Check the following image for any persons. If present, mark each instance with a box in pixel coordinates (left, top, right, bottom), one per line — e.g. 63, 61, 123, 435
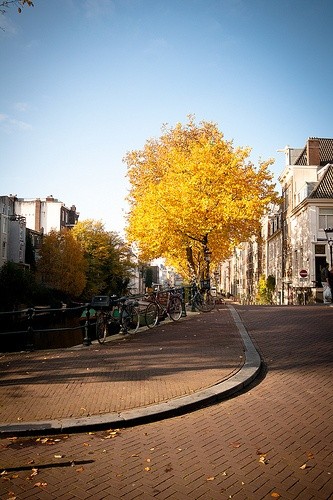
318, 263, 333, 304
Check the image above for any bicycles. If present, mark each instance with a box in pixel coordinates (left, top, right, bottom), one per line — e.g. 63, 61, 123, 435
144, 284, 183, 329
187, 282, 215, 312
91, 294, 141, 344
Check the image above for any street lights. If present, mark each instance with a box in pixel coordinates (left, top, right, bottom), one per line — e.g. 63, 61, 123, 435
323, 227, 333, 270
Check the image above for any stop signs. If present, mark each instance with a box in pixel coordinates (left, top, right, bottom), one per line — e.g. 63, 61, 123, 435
300, 270, 308, 277
